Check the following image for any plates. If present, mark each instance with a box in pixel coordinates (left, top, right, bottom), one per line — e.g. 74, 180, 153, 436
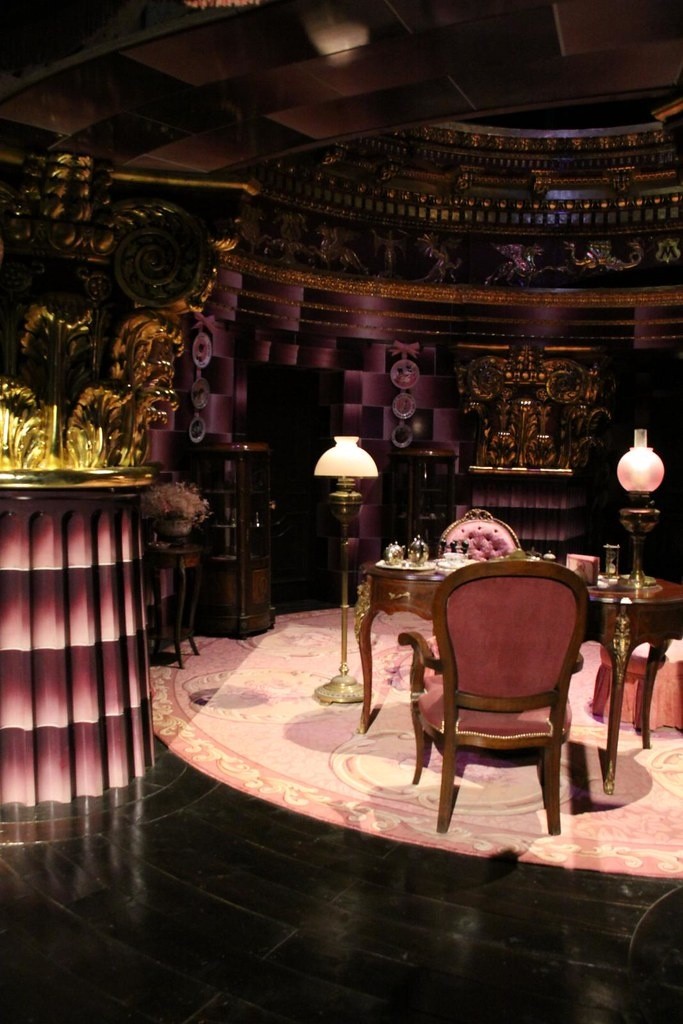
193, 333, 212, 368
391, 424, 413, 448
392, 393, 417, 419
436, 561, 471, 568
189, 417, 205, 443
390, 360, 420, 389
191, 378, 209, 409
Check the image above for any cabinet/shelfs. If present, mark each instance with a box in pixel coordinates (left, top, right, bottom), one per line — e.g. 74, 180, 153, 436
202, 442, 272, 634
387, 449, 457, 545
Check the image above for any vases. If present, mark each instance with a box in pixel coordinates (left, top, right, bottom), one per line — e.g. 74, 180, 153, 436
157, 518, 191, 537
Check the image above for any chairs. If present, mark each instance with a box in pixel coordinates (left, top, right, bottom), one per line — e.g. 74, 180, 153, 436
398, 560, 588, 835
589, 639, 683, 731
437, 509, 521, 561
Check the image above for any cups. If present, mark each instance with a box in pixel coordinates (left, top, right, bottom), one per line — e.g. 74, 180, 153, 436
444, 552, 467, 566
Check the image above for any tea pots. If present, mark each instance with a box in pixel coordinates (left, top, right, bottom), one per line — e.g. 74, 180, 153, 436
407, 535, 429, 565
384, 542, 405, 566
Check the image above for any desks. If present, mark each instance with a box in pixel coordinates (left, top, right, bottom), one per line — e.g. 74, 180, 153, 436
353, 556, 683, 795
146, 545, 202, 669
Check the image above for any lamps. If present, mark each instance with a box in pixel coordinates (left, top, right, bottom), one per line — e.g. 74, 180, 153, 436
314, 436, 379, 703
616, 429, 664, 585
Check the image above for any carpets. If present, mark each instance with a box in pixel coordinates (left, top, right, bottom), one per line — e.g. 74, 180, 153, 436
148, 607, 683, 877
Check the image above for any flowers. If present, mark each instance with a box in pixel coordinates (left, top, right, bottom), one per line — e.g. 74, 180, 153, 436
140, 482, 209, 519
